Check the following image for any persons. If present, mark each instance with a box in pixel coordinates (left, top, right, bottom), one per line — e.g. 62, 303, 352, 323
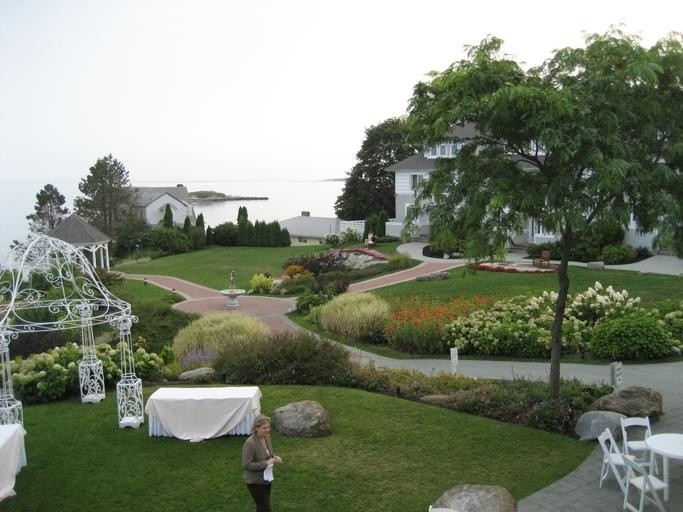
240, 413, 282, 511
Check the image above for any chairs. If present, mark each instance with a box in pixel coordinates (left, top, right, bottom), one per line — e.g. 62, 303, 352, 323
620, 416, 659, 475
597, 428, 635, 494
533, 250, 550, 267
622, 454, 669, 512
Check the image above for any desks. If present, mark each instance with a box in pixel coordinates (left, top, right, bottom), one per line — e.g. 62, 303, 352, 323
646, 433, 683, 501
0, 423, 27, 500
145, 386, 263, 443
